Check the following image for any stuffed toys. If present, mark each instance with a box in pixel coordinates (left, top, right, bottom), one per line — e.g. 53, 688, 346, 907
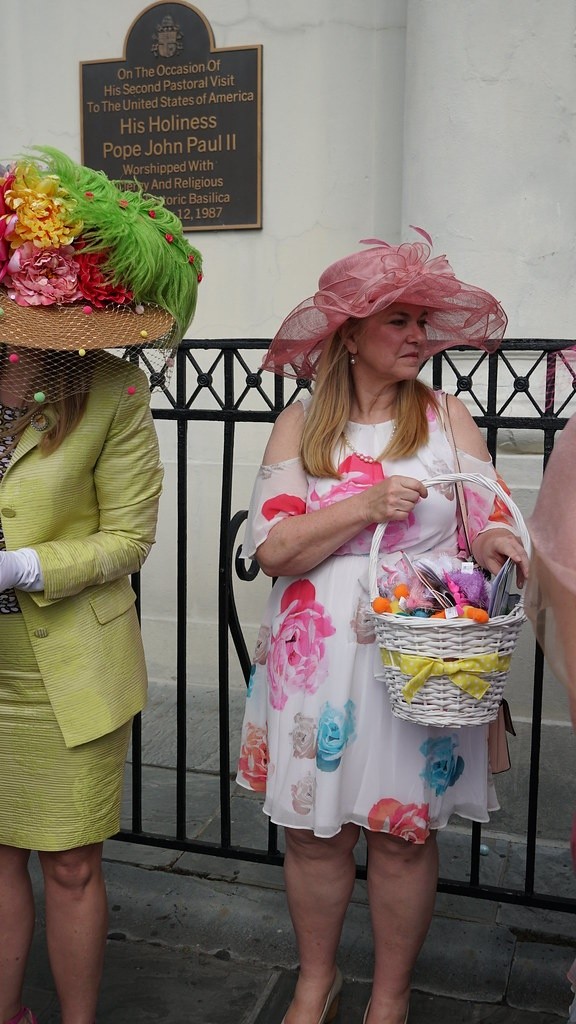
371, 563, 489, 623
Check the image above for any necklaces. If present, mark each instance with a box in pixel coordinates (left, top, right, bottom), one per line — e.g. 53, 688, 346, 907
343, 425, 397, 464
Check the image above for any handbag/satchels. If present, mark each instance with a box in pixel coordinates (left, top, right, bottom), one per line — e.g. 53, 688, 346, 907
488, 699, 516, 774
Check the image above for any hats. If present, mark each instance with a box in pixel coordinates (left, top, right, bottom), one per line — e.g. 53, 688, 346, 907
0, 145, 203, 403
257, 225, 508, 382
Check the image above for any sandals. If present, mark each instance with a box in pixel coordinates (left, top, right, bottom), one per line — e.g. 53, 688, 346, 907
4, 1004, 38, 1024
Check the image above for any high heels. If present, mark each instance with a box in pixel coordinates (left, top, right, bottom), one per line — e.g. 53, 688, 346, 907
362, 995, 409, 1024
281, 965, 342, 1024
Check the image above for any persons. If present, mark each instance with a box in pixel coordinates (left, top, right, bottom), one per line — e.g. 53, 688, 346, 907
236, 241, 531, 1024
0, 145, 201, 1024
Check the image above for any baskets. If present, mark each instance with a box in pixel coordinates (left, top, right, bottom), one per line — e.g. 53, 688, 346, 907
365, 475, 531, 728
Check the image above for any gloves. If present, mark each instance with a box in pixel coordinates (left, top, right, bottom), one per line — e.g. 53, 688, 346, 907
0, 547, 44, 593
